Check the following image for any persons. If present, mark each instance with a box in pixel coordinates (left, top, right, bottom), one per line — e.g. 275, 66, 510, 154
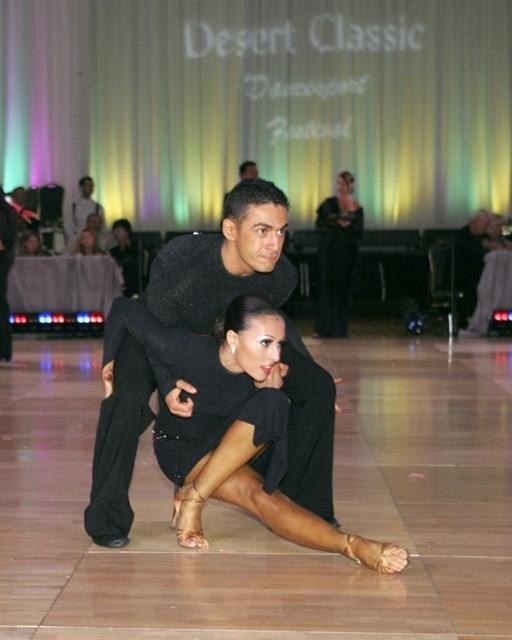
482, 211, 511, 249
236, 159, 260, 179
0, 174, 152, 372
99, 291, 412, 577
311, 168, 365, 339
438, 208, 511, 342
82, 175, 344, 549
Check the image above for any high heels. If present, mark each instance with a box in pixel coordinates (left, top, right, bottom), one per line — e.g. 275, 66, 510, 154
169, 480, 209, 549
342, 532, 410, 575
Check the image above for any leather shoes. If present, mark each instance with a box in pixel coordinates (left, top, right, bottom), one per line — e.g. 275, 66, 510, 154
107, 537, 130, 548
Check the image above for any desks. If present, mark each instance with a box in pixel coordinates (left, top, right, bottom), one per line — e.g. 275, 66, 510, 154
289, 246, 412, 303
7, 255, 123, 321
473, 250, 512, 335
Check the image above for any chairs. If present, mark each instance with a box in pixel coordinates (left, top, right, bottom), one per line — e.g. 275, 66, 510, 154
423, 229, 469, 335
